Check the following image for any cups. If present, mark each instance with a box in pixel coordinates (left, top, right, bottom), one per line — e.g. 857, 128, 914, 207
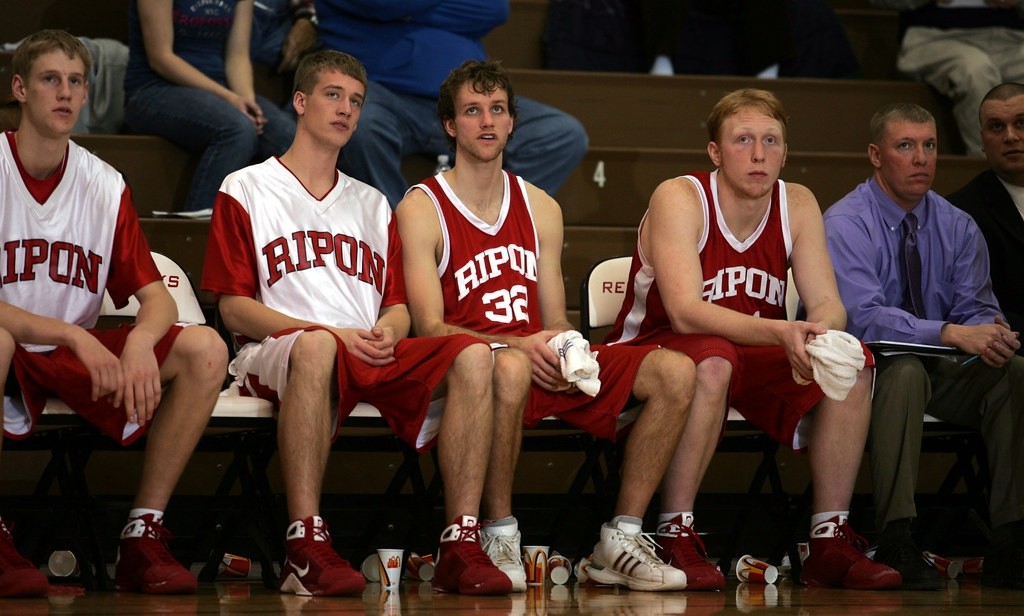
378, 549, 404, 588
48, 550, 76, 576
360, 553, 381, 582
405, 552, 435, 580
735, 555, 778, 583
547, 555, 572, 584
959, 557, 984, 574
524, 546, 549, 585
922, 550, 959, 578
209, 550, 250, 580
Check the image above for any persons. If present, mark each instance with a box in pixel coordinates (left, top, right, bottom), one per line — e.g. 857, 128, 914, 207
894, 1, 1024, 161
394, 59, 687, 593
822, 101, 1024, 589
201, 51, 512, 596
603, 87, 902, 591
943, 84, 1024, 338
122, 0, 590, 218
0, 26, 229, 598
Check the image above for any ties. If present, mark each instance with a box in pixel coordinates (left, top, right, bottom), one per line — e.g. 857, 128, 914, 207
901, 212, 928, 320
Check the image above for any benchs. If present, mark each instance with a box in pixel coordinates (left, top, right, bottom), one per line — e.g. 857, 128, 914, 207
0, 0, 992, 345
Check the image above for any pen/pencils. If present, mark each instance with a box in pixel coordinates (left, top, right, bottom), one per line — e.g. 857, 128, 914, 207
959, 354, 980, 367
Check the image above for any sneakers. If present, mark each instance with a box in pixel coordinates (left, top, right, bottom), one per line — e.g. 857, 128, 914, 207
433, 515, 513, 596
801, 514, 903, 590
586, 591, 687, 616
277, 516, 367, 596
115, 513, 197, 595
0, 520, 49, 597
655, 512, 726, 589
586, 515, 687, 591
480, 517, 527, 592
872, 536, 945, 589
979, 536, 1024, 590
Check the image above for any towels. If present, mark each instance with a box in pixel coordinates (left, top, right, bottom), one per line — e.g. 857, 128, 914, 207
790, 328, 866, 402
547, 328, 603, 397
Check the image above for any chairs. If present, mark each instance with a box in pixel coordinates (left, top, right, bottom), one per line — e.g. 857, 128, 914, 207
0, 249, 1024, 589
